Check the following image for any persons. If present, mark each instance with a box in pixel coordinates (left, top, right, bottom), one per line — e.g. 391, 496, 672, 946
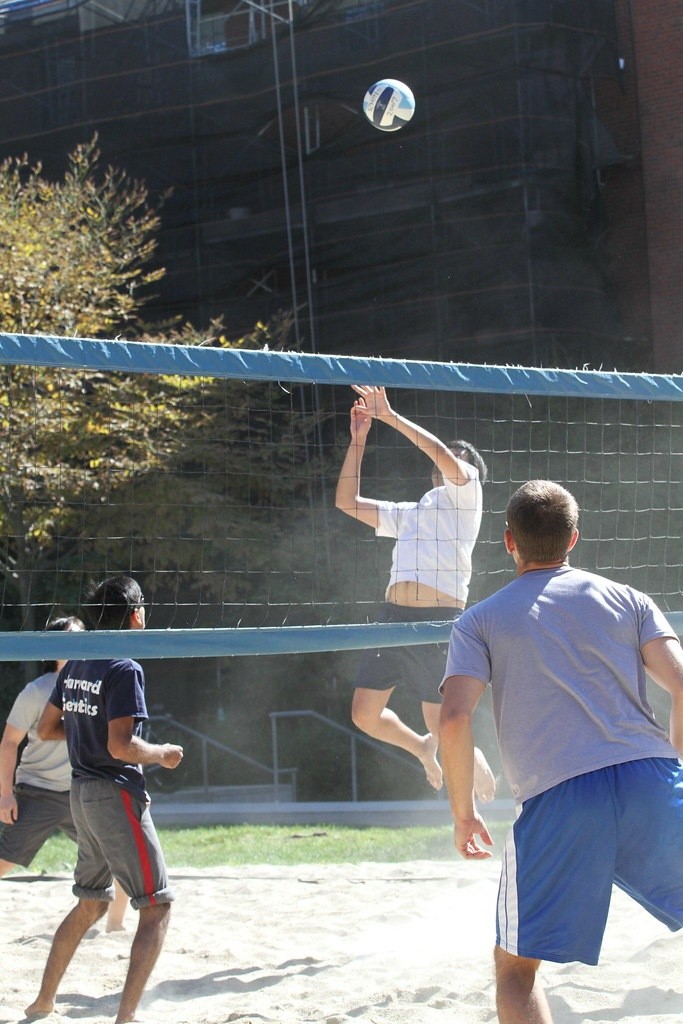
440, 479, 683, 1023
23, 574, 183, 1023
336, 386, 496, 808
0, 616, 129, 931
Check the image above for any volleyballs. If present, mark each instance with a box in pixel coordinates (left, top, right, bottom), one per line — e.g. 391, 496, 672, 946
361, 78, 417, 133
